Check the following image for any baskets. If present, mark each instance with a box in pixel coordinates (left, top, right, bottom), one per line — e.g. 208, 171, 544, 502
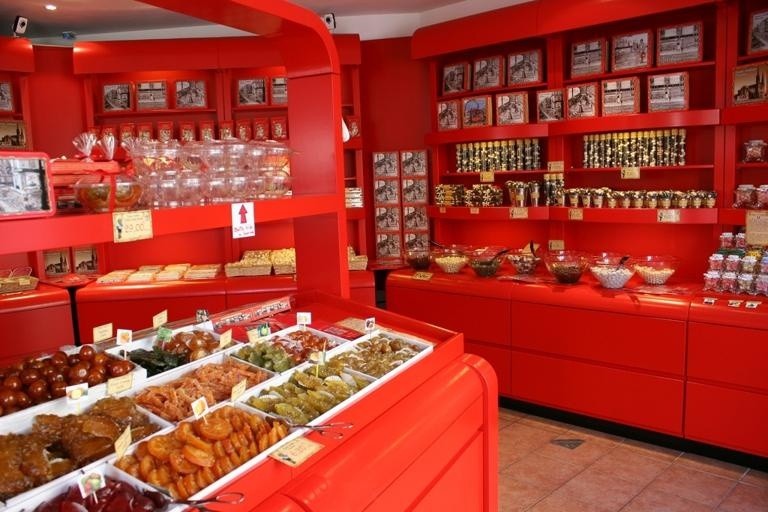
0, 266, 40, 294
224, 246, 368, 278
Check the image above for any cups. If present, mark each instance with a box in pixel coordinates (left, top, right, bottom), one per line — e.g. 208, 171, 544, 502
507, 183, 541, 209
567, 190, 717, 210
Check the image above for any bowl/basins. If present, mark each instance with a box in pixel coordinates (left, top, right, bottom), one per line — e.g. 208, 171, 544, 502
403, 243, 680, 290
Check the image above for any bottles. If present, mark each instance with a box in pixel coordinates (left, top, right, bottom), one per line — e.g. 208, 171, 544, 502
435, 183, 505, 208
743, 138, 766, 162
73, 136, 293, 211
455, 139, 542, 170
543, 172, 565, 207
735, 184, 768, 209
705, 231, 767, 295
582, 128, 686, 168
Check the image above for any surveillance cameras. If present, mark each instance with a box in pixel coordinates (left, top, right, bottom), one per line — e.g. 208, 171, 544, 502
12, 15, 29, 37
322, 13, 337, 31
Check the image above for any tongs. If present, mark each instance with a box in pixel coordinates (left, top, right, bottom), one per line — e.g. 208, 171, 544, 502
265, 410, 355, 439
142, 484, 246, 512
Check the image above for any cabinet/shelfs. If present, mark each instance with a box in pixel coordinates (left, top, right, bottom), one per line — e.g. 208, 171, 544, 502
386, 259, 511, 397
510, 270, 706, 444
1, 291, 499, 512
2, 71, 77, 361
685, 288, 767, 460
410, 1, 768, 279
74, 38, 377, 345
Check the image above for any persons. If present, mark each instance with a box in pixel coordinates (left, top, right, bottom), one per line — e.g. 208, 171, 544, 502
634, 38, 646, 63
